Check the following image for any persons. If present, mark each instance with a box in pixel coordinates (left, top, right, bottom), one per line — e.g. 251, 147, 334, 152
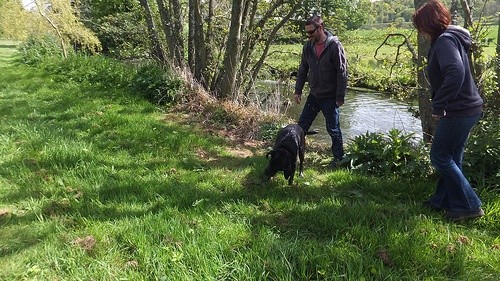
412, 0, 484, 221
295, 15, 351, 168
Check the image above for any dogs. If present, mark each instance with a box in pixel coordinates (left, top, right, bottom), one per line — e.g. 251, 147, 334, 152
262, 124, 319, 186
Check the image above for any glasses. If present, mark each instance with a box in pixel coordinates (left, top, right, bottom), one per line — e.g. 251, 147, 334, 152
306, 26, 319, 36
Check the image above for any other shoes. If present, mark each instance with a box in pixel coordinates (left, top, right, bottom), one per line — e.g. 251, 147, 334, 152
444, 208, 485, 221
327, 160, 337, 170
423, 198, 442, 211
